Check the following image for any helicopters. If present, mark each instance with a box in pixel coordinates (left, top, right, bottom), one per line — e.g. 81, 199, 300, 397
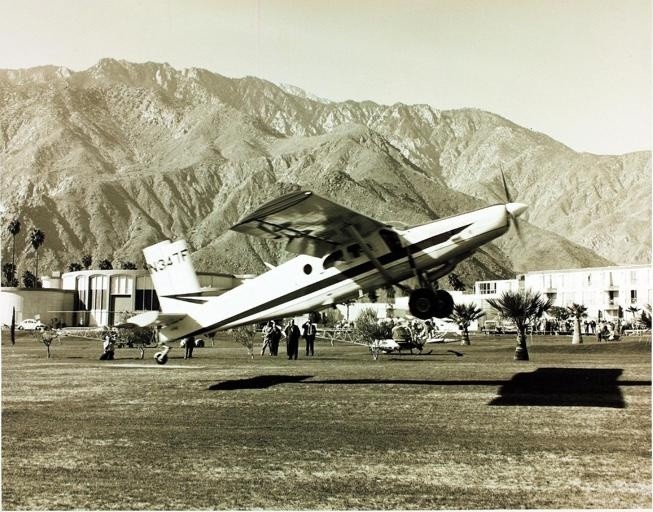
46, 309, 156, 348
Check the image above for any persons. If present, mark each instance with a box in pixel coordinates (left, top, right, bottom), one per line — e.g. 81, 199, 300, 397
183, 336, 195, 359
302, 319, 317, 356
266, 322, 281, 356
331, 315, 435, 341
51, 317, 60, 330
285, 319, 301, 360
527, 316, 628, 343
281, 321, 291, 356
99, 332, 115, 360
261, 321, 272, 355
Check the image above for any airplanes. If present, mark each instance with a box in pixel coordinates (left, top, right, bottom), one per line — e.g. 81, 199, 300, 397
315, 317, 465, 360
127, 164, 529, 365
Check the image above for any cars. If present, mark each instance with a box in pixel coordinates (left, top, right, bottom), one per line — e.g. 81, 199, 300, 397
17, 318, 46, 330
481, 320, 496, 334
